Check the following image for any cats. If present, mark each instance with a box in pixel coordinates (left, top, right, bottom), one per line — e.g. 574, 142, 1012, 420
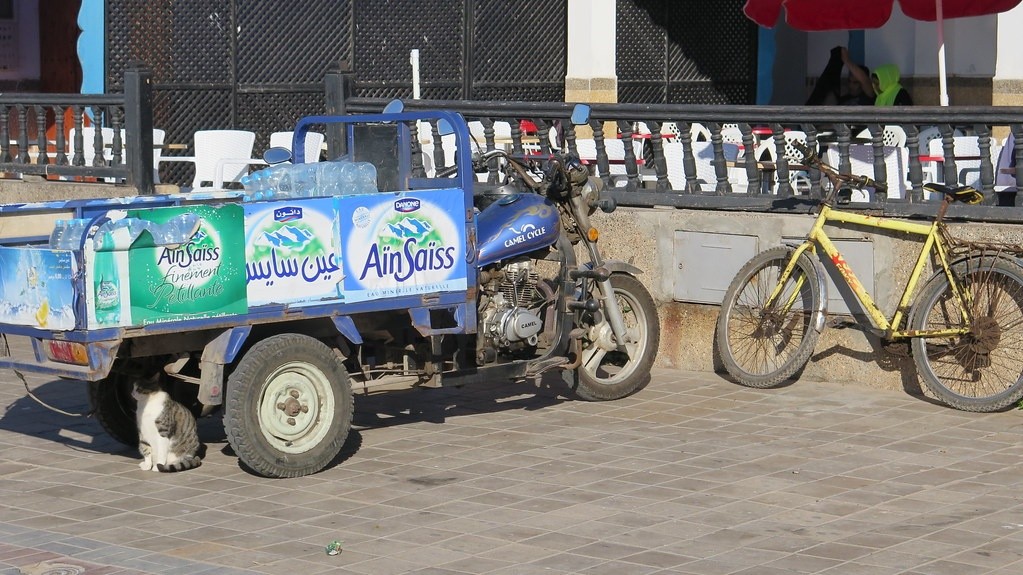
131, 372, 201, 473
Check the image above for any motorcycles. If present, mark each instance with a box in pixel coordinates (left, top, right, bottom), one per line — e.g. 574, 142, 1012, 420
0, 102, 662, 480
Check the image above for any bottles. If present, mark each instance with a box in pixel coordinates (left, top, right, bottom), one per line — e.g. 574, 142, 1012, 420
242, 162, 378, 203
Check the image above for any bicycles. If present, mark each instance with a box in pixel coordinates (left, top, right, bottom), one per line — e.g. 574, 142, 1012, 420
713, 161, 1023, 419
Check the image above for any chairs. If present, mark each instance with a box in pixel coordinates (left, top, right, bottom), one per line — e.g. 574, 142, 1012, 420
60, 121, 1016, 204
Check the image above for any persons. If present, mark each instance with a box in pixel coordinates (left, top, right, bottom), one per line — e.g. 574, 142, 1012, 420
807, 47, 920, 159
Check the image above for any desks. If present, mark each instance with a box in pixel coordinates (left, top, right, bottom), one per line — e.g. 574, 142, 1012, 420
578, 159, 647, 176
9, 139, 69, 147
709, 158, 830, 196
471, 136, 540, 153
751, 128, 794, 145
615, 133, 675, 143
917, 155, 981, 199
104, 142, 188, 150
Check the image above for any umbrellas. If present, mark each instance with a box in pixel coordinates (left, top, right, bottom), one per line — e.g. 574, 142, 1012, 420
744, 0, 1022, 106
